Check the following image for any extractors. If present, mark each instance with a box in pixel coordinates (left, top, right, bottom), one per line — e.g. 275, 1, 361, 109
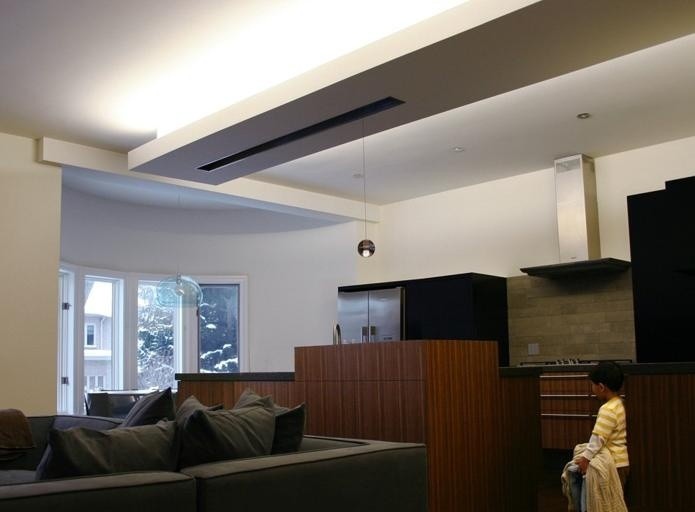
520, 153, 632, 283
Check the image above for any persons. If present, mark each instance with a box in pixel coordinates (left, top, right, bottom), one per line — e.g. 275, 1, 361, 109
575, 361, 633, 512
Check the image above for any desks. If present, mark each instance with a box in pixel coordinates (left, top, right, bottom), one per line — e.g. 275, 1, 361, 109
103, 389, 178, 404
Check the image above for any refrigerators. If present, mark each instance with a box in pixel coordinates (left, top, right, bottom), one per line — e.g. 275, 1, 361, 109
334, 285, 404, 344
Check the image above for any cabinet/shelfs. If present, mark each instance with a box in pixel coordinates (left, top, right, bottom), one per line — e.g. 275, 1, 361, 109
538, 364, 627, 452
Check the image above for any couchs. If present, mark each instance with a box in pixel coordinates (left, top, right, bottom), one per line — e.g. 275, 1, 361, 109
0, 413, 433, 511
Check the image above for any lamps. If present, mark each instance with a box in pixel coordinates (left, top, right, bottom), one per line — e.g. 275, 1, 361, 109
155, 187, 204, 310
354, 107, 376, 260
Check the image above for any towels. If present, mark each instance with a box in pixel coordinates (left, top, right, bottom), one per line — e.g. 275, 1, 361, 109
0, 408, 38, 462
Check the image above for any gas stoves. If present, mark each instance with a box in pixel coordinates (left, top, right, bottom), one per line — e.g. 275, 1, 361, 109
509, 358, 634, 374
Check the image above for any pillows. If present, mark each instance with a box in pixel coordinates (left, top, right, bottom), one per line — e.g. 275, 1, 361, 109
175, 394, 225, 428
229, 386, 309, 452
116, 387, 180, 428
177, 403, 279, 467
31, 424, 181, 483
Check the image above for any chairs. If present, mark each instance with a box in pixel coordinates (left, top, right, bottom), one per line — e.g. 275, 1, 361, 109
83, 390, 113, 418
